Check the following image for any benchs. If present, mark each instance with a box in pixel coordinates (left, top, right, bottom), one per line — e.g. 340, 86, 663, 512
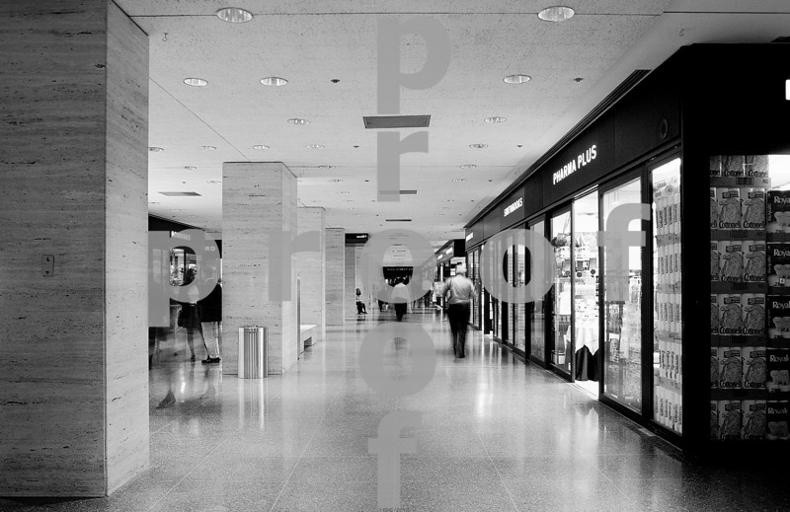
300, 322, 318, 354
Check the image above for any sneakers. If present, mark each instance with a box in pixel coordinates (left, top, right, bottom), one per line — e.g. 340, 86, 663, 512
201, 355, 220, 364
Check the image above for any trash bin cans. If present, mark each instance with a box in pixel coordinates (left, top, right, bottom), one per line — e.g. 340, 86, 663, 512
238, 326, 266, 379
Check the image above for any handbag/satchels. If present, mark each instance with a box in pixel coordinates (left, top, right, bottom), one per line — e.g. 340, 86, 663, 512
176, 306, 202, 329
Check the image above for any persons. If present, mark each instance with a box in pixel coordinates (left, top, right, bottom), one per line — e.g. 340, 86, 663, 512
354, 279, 447, 320
442, 263, 479, 360
181, 268, 208, 363
198, 283, 225, 365
